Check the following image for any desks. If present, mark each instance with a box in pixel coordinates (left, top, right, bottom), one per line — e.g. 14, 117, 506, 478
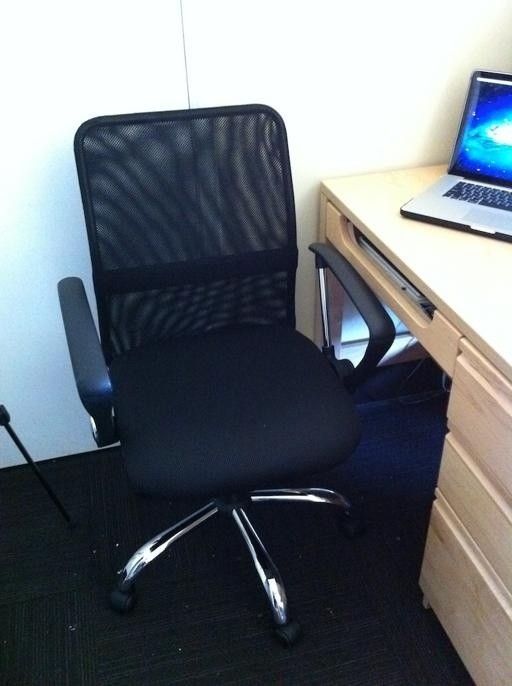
313, 165, 512, 686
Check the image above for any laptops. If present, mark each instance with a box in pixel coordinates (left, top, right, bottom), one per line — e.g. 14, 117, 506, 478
400, 70, 512, 243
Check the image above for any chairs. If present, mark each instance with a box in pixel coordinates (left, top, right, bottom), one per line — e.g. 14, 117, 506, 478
57, 105, 395, 646
